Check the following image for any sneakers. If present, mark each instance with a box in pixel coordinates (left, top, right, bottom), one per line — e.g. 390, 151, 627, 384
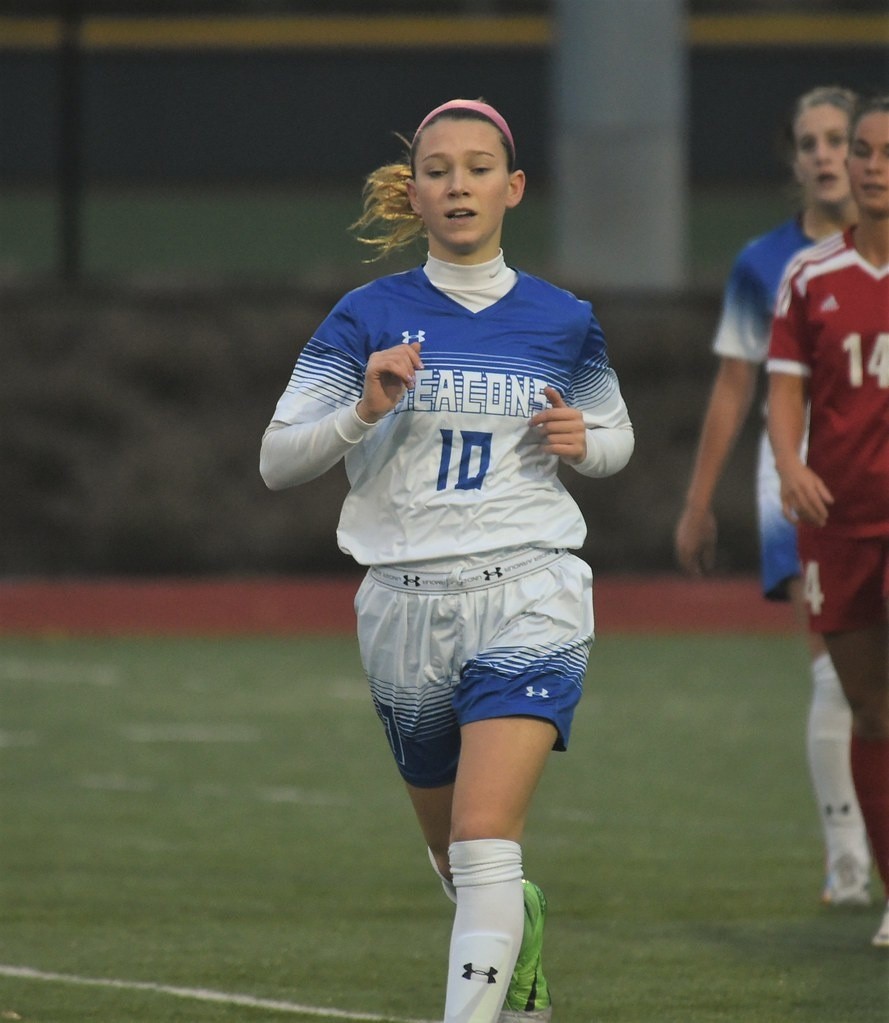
498, 879, 553, 1023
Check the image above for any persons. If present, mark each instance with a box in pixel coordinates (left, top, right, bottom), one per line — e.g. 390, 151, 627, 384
259, 101, 635, 1023
675, 90, 889, 953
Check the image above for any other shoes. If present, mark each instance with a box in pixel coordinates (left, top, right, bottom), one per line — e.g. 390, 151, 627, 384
872, 912, 889, 948
822, 839, 873, 907
